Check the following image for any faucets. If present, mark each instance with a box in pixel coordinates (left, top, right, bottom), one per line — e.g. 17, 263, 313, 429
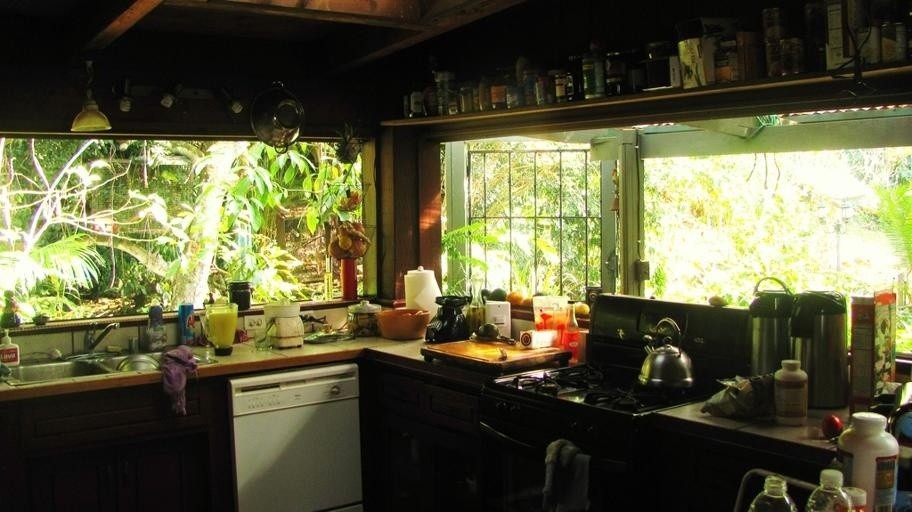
82, 321, 121, 352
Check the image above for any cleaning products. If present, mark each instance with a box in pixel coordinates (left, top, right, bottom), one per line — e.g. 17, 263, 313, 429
0, 328, 21, 367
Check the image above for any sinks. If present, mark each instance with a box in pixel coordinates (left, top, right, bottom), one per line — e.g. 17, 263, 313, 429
99, 344, 217, 372
1, 358, 115, 387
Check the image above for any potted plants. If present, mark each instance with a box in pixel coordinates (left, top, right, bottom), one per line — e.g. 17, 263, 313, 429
425, 220, 498, 342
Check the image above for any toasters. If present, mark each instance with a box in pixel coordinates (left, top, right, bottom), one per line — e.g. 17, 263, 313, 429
486, 301, 512, 339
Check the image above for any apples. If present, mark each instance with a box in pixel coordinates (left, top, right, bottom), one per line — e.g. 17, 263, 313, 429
820, 413, 844, 439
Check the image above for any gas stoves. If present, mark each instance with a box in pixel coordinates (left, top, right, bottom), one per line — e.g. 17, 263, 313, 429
495, 361, 701, 416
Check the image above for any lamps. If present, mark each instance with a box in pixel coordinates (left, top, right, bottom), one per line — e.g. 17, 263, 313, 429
69, 60, 113, 133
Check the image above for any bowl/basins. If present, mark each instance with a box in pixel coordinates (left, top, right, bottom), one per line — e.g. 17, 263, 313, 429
117, 354, 160, 371
376, 310, 430, 340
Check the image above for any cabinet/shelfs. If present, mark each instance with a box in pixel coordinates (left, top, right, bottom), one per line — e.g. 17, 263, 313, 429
0, 380, 212, 512
363, 359, 480, 512
641, 426, 821, 512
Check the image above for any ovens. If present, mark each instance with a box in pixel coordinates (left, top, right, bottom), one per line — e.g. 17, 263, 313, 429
477, 418, 635, 512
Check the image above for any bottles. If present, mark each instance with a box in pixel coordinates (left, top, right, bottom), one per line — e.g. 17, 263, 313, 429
888, 381, 912, 447
562, 299, 580, 364
747, 412, 900, 512
348, 300, 381, 338
229, 281, 251, 310
129, 335, 139, 353
466, 274, 484, 337
774, 359, 809, 427
406, 41, 604, 119
179, 303, 196, 346
145, 305, 168, 353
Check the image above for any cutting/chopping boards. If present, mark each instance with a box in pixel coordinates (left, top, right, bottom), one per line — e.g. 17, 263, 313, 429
420, 337, 573, 375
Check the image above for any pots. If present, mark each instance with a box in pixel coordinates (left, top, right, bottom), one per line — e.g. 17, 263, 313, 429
249, 82, 306, 154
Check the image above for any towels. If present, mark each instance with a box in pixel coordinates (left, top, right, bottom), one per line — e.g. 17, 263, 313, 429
541, 437, 593, 512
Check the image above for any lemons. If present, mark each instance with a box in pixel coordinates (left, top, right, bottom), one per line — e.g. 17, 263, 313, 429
520, 296, 534, 306
479, 289, 490, 303
505, 291, 522, 307
571, 300, 590, 316
489, 287, 506, 302
478, 324, 499, 340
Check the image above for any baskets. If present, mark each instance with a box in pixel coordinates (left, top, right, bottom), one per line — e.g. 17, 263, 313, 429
326, 221, 376, 259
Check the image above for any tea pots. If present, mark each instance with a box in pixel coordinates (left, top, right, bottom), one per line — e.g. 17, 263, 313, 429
637, 317, 697, 391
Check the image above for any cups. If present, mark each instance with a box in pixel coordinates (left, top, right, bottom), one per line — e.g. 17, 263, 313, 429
254, 329, 272, 351
204, 302, 240, 349
